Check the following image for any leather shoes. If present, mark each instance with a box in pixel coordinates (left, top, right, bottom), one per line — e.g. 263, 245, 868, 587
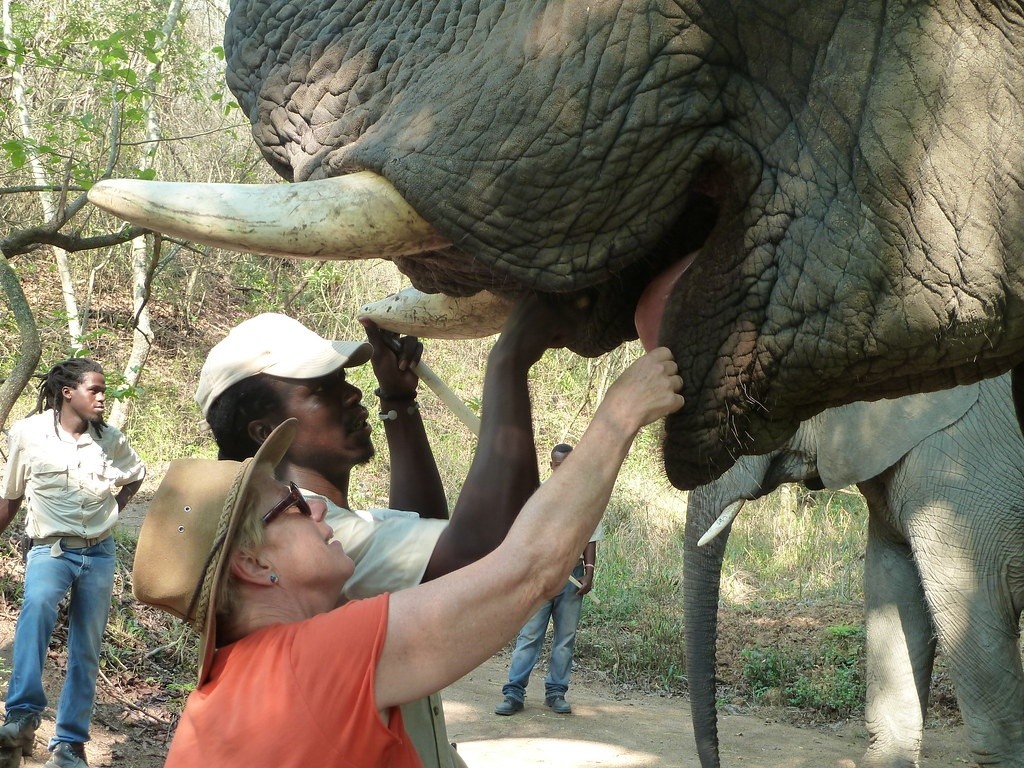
545, 695, 571, 713
495, 697, 523, 715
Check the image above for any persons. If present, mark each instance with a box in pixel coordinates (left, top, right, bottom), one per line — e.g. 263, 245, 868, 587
0, 359, 146, 768
132, 313, 684, 768
494, 444, 602, 716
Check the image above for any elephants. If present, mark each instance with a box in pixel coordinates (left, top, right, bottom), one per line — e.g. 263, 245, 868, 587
86, 1, 1023, 768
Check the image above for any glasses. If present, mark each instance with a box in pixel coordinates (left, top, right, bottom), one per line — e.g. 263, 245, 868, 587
238, 481, 312, 547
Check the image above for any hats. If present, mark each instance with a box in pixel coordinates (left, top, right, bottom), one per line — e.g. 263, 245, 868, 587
131, 417, 298, 689
194, 312, 374, 433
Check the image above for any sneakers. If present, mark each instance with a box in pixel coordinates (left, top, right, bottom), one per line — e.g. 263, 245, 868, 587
0, 710, 42, 756
45, 742, 88, 768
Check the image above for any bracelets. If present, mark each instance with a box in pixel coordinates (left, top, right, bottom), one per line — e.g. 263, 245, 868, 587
585, 564, 594, 568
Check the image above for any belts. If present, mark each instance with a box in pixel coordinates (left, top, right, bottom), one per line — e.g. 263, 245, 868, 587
33, 528, 113, 558
